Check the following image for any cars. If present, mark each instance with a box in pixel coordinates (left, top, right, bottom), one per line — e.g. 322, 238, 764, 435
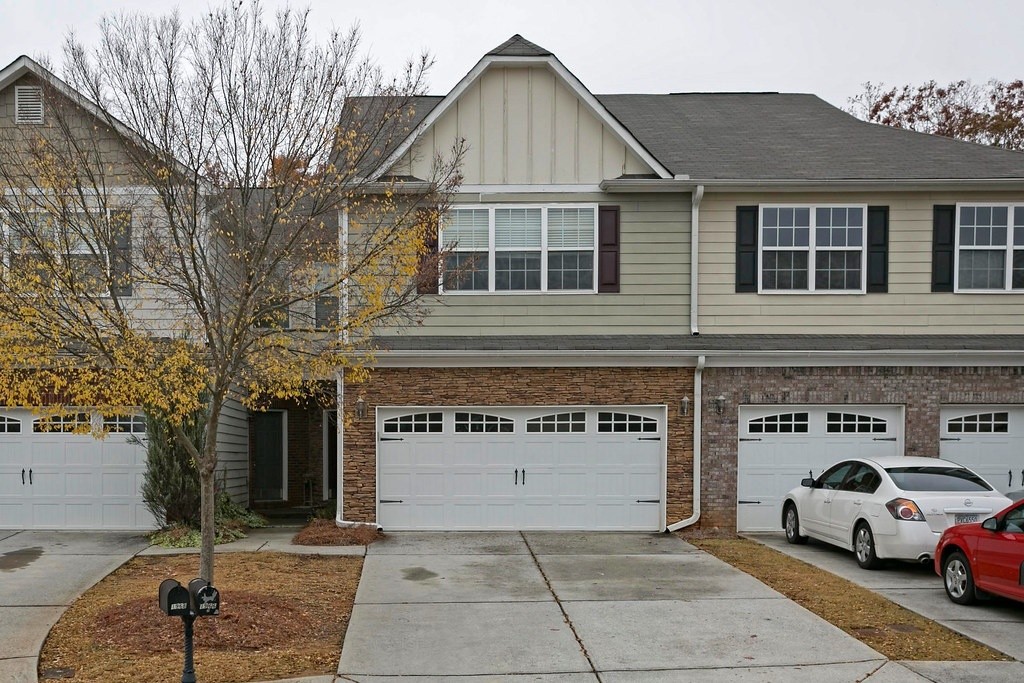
779, 455, 1014, 569
933, 498, 1024, 605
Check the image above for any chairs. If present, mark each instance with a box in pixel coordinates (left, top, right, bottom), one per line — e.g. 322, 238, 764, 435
861, 473, 876, 487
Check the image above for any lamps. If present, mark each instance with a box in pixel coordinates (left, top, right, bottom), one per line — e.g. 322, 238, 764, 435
715, 395, 726, 416
351, 395, 369, 428
677, 392, 690, 417
